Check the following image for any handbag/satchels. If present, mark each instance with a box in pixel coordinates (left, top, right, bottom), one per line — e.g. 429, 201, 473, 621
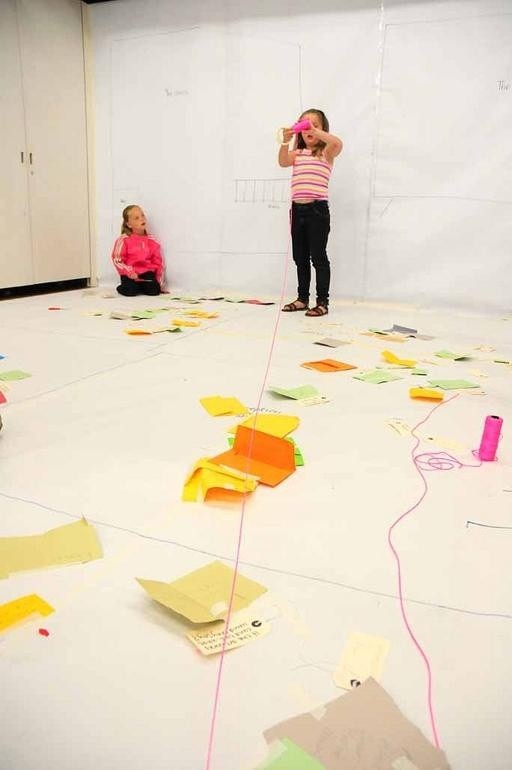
281, 141, 291, 146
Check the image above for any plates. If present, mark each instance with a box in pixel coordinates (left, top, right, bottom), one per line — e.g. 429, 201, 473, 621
0, 3, 98, 291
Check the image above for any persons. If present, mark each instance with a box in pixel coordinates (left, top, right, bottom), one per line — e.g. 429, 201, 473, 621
278, 108, 343, 317
111, 205, 171, 296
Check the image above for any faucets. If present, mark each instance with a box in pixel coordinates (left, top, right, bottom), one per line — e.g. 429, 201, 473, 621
282, 298, 328, 316
